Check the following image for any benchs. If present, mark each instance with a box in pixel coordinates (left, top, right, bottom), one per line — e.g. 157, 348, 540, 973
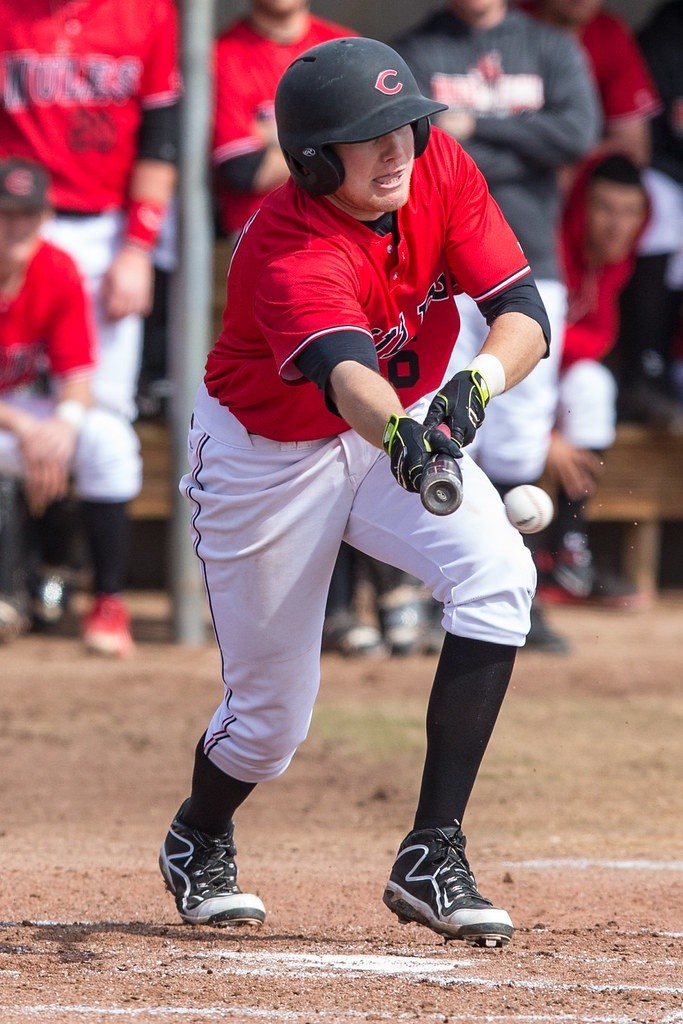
130, 416, 683, 613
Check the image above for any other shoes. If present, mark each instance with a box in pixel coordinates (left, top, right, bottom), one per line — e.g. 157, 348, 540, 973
323, 589, 574, 659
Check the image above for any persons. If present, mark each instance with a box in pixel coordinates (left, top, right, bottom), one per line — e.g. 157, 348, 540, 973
508, 0, 657, 195
1, 0, 185, 425
548, 144, 653, 598
158, 36, 555, 948
383, 0, 599, 657
208, 0, 361, 245
0, 158, 144, 658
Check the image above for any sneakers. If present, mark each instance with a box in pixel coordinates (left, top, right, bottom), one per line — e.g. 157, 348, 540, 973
81, 597, 134, 659
159, 797, 266, 926
537, 558, 636, 609
382, 826, 514, 946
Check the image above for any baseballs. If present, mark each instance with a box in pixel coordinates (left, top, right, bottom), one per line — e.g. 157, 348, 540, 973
506, 485, 555, 535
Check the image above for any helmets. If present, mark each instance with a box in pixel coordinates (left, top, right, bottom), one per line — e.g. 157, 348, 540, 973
273, 36, 449, 196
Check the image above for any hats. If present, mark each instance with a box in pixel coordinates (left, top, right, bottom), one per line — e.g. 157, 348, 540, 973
0, 166, 45, 211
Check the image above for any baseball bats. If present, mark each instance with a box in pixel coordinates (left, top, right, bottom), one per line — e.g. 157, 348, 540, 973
419, 422, 465, 517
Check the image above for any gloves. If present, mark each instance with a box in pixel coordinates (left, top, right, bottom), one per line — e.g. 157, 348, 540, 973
381, 413, 464, 492
423, 369, 492, 449
